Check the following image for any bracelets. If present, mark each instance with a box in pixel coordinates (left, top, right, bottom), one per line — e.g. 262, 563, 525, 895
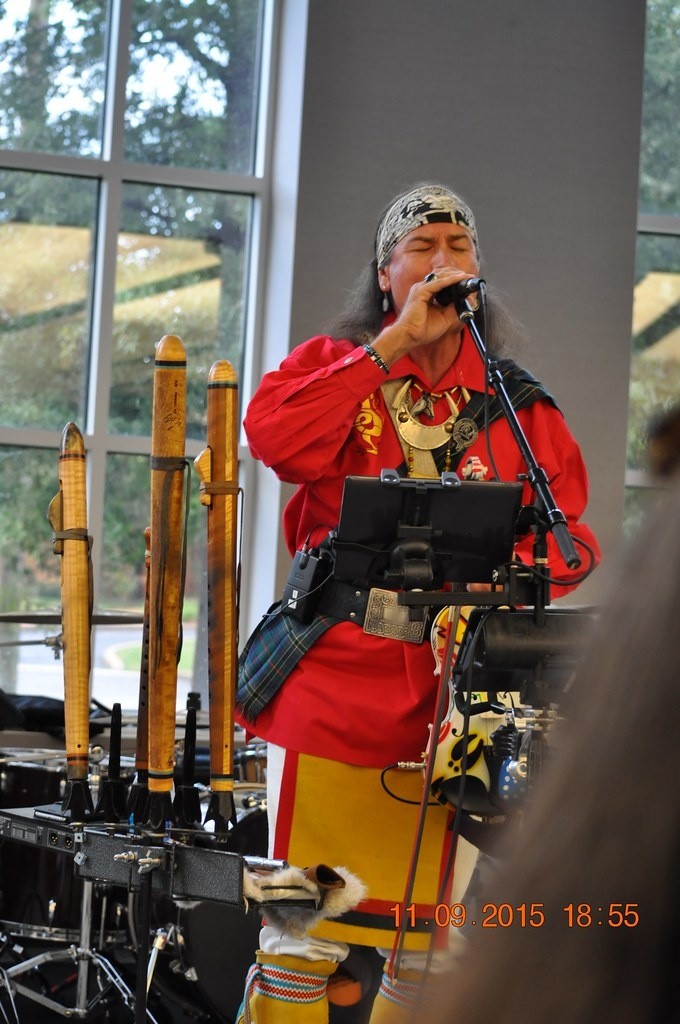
362, 343, 390, 377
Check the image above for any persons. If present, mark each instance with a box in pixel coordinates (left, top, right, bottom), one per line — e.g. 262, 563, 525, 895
237, 182, 599, 1024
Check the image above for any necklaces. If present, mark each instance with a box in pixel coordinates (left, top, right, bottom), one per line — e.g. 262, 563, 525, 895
403, 442, 454, 481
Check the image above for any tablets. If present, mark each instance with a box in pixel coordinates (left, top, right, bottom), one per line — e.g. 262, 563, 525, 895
332, 475, 524, 588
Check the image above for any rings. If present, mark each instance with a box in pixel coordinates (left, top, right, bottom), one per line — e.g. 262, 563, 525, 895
424, 272, 436, 282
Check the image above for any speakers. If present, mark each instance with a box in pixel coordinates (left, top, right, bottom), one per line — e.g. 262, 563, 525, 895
0, 802, 269, 1024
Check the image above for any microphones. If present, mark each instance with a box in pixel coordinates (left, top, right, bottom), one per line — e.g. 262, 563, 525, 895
432, 278, 489, 306
